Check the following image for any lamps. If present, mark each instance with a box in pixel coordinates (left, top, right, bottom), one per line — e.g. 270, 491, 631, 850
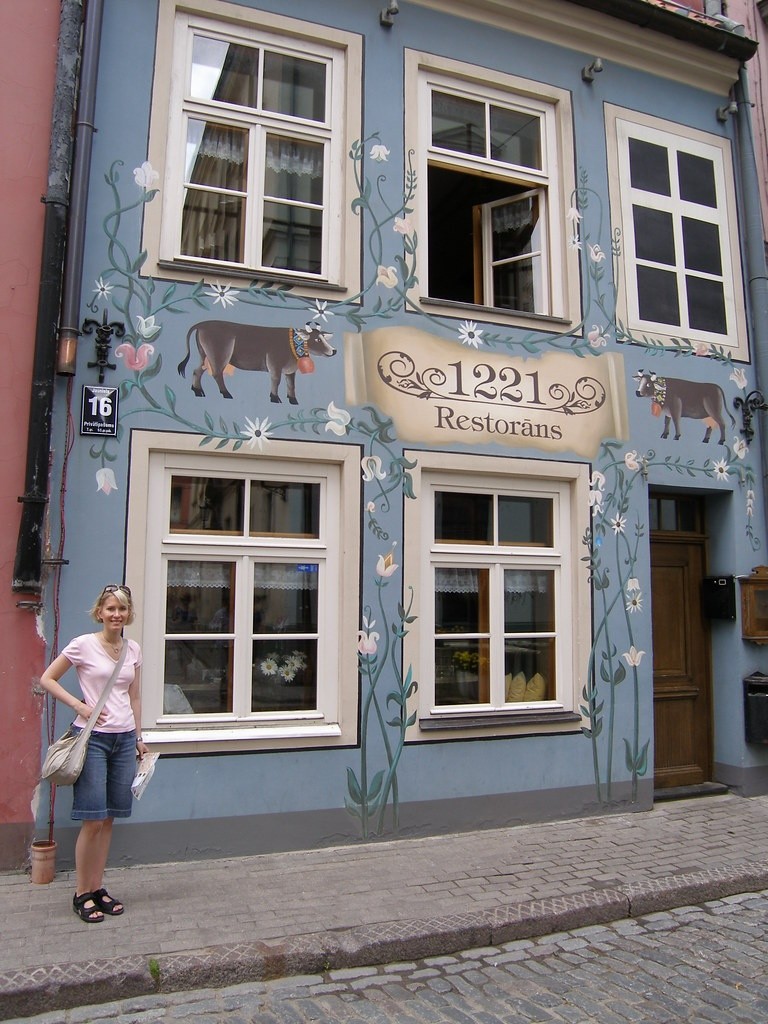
716, 100, 738, 122
582, 58, 603, 82
379, 0, 400, 27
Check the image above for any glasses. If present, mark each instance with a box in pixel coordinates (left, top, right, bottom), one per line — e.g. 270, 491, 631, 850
100, 585, 131, 600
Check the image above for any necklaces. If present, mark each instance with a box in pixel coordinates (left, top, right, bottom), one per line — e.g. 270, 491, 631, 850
101, 633, 121, 653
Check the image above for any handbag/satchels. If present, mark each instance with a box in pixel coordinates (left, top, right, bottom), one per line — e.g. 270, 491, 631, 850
42, 731, 91, 786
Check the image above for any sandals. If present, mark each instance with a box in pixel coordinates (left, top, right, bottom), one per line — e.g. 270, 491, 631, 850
72, 892, 104, 922
92, 888, 124, 915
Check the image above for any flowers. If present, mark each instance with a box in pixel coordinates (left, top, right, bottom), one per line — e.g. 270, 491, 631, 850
452, 650, 486, 673
260, 649, 308, 683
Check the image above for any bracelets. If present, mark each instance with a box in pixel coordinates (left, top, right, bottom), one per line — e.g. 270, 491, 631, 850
136, 737, 143, 742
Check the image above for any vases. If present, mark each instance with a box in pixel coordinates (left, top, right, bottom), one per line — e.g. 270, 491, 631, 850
455, 671, 483, 699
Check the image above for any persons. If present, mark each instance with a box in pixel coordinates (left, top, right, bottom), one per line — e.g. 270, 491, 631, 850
40, 584, 149, 923
